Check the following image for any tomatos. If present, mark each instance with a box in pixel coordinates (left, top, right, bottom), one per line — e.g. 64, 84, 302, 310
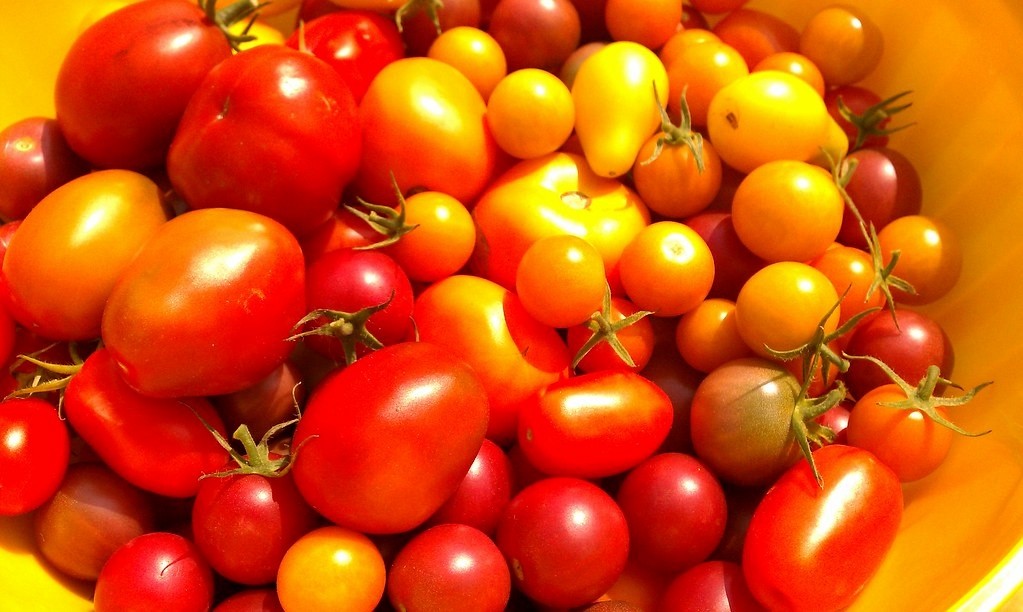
0, 0, 961, 612
